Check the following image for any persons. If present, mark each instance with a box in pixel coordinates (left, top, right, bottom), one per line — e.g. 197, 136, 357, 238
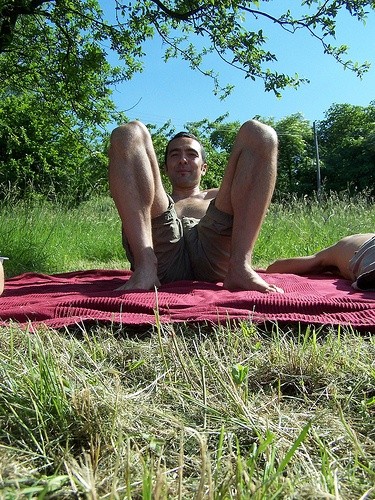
255, 233, 375, 291
108, 120, 284, 294
